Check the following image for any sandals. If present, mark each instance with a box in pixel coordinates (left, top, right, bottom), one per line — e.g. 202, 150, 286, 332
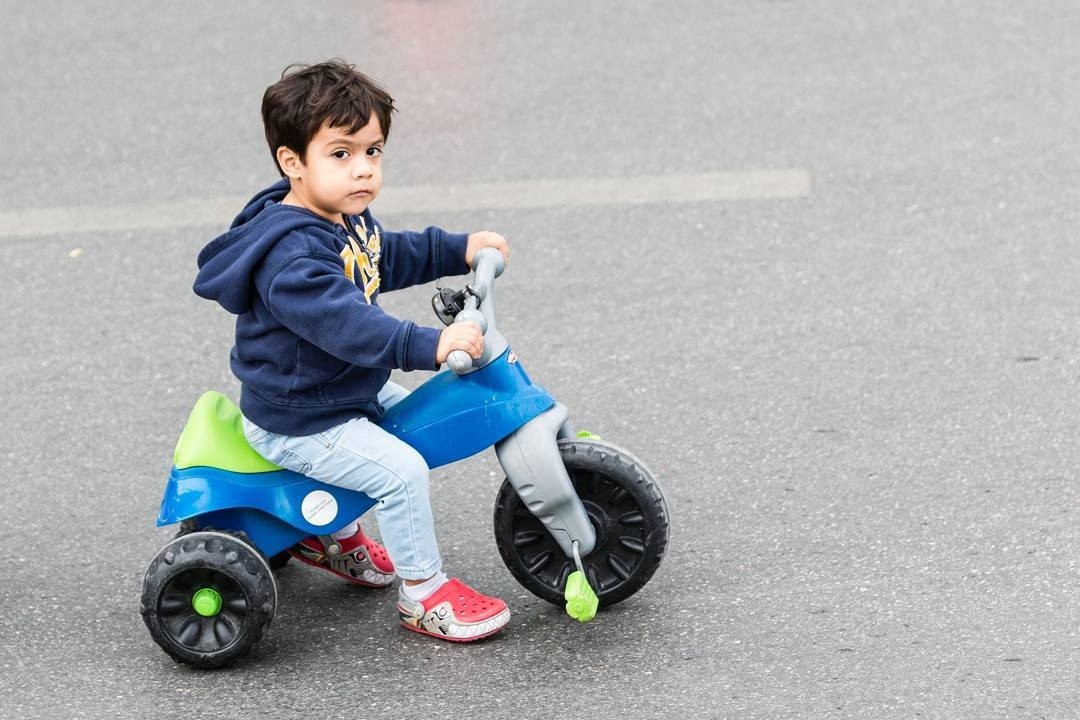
287, 521, 396, 588
398, 578, 512, 641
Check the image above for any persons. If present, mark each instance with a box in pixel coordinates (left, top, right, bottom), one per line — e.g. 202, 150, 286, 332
193, 58, 512, 642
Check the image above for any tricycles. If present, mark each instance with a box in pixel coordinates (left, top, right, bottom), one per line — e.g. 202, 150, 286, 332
138, 242, 673, 668
